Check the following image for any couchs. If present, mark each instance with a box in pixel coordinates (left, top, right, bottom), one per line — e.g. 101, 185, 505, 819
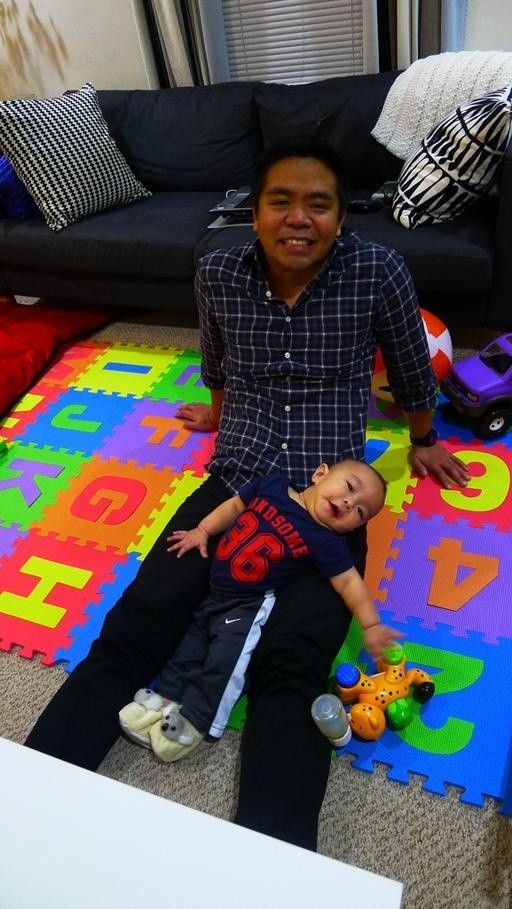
0, 62, 511, 332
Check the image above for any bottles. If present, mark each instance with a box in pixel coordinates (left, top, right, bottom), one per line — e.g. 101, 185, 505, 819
311, 694, 352, 748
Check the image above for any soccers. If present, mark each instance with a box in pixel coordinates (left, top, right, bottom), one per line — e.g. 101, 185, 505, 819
372, 309, 454, 401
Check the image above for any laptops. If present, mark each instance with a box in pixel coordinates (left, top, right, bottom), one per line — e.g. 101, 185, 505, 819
207, 108, 342, 220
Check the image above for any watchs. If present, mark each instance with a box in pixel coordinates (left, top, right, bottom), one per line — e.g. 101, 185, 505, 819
409, 429, 439, 447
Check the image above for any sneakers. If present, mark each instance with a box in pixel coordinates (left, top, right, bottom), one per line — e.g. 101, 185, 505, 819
119, 688, 203, 763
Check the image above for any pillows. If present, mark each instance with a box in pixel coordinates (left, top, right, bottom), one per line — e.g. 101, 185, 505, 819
0, 82, 152, 232
393, 85, 510, 230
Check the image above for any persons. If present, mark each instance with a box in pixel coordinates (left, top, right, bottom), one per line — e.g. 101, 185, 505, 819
21, 137, 471, 851
118, 458, 407, 762
